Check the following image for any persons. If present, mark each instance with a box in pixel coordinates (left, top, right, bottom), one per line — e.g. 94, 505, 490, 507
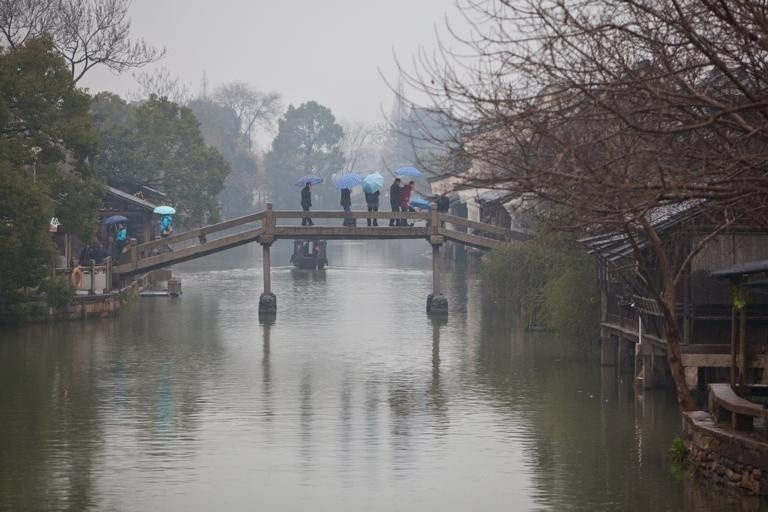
340, 188, 352, 226
400, 181, 415, 226
79, 243, 106, 266
304, 241, 309, 255
365, 190, 381, 226
389, 178, 404, 226
112, 223, 127, 266
157, 215, 174, 255
436, 193, 449, 228
301, 182, 315, 226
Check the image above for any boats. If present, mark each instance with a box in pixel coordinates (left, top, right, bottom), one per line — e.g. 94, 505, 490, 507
287, 238, 328, 269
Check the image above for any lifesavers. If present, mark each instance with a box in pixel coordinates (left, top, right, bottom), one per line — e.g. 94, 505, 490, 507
72, 268, 83, 290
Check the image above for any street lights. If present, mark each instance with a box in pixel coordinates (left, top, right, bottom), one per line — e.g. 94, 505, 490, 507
30, 144, 43, 184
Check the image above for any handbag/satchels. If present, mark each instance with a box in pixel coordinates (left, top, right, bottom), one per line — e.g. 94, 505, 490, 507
160, 224, 174, 236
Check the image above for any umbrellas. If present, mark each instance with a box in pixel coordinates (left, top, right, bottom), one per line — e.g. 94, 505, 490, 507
152, 205, 177, 221
393, 167, 423, 177
294, 175, 323, 192
362, 173, 384, 194
410, 199, 432, 209
100, 215, 129, 227
335, 174, 362, 192
